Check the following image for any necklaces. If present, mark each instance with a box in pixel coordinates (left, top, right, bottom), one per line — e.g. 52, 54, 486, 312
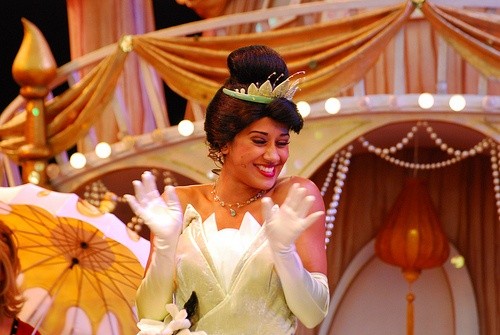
211, 182, 266, 216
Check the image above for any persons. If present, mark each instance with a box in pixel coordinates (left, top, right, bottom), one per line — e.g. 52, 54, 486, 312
124, 47, 328, 335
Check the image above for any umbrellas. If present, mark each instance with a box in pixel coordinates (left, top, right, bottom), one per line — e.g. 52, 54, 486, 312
0, 184, 152, 335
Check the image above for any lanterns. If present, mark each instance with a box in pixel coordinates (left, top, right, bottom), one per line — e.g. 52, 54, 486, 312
374, 176, 449, 335
